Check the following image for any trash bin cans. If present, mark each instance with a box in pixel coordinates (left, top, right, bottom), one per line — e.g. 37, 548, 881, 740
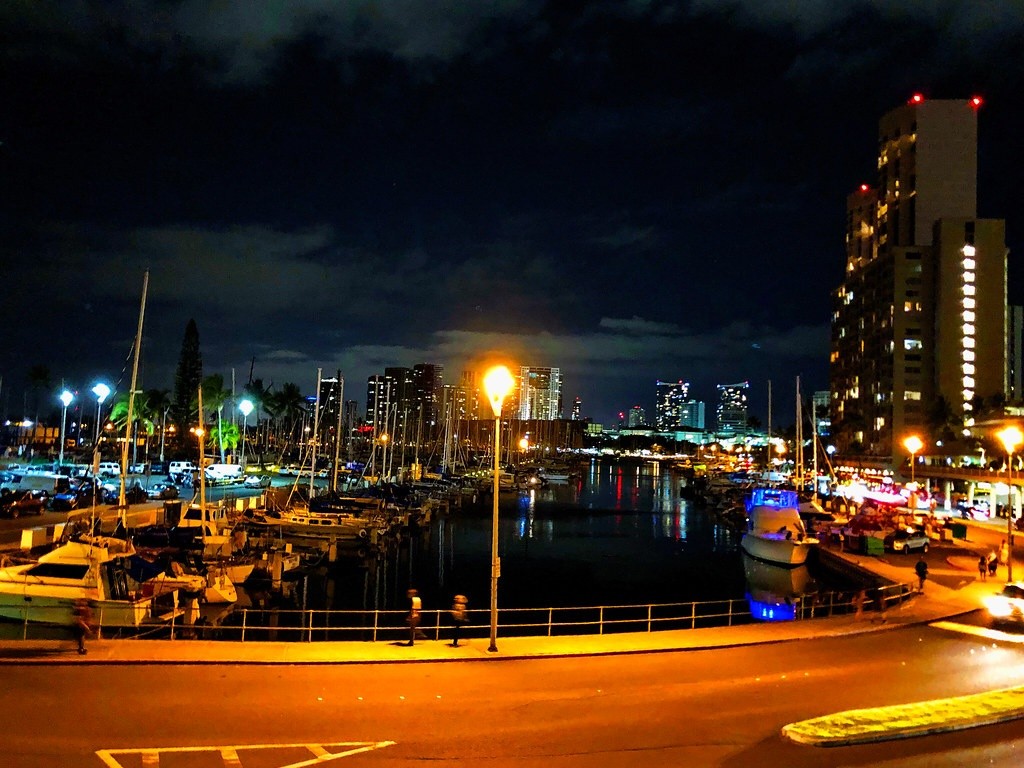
859, 534, 884, 556
943, 523, 967, 540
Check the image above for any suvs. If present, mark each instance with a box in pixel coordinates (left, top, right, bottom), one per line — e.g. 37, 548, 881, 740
295, 468, 317, 477
346, 474, 365, 484
0, 488, 46, 517
279, 466, 297, 474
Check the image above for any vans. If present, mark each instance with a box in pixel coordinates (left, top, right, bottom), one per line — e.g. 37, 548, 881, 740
170, 461, 197, 474
97, 461, 121, 478
0, 471, 70, 496
206, 463, 244, 477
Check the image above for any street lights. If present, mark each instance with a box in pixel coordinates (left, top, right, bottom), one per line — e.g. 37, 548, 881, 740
483, 365, 520, 651
904, 436, 923, 521
999, 424, 1022, 581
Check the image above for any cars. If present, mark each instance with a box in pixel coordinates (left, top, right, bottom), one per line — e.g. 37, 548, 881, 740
244, 473, 272, 489
994, 584, 1024, 626
338, 472, 350, 482
150, 464, 164, 474
8, 463, 35, 471
145, 481, 178, 499
50, 480, 98, 510
103, 474, 145, 505
181, 469, 224, 487
315, 469, 328, 478
70, 478, 90, 493
887, 529, 932, 554
130, 463, 147, 474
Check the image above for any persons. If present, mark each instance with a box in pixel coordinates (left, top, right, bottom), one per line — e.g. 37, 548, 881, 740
73, 601, 91, 654
839, 528, 845, 552
987, 552, 997, 575
405, 589, 427, 646
915, 557, 928, 590
999, 539, 1009, 566
978, 555, 987, 579
449, 595, 470, 646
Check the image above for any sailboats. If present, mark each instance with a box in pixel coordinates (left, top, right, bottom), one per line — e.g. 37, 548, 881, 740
0, 274, 580, 630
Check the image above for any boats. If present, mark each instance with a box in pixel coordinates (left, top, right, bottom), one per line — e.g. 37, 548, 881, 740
739, 488, 821, 566
674, 447, 832, 531
